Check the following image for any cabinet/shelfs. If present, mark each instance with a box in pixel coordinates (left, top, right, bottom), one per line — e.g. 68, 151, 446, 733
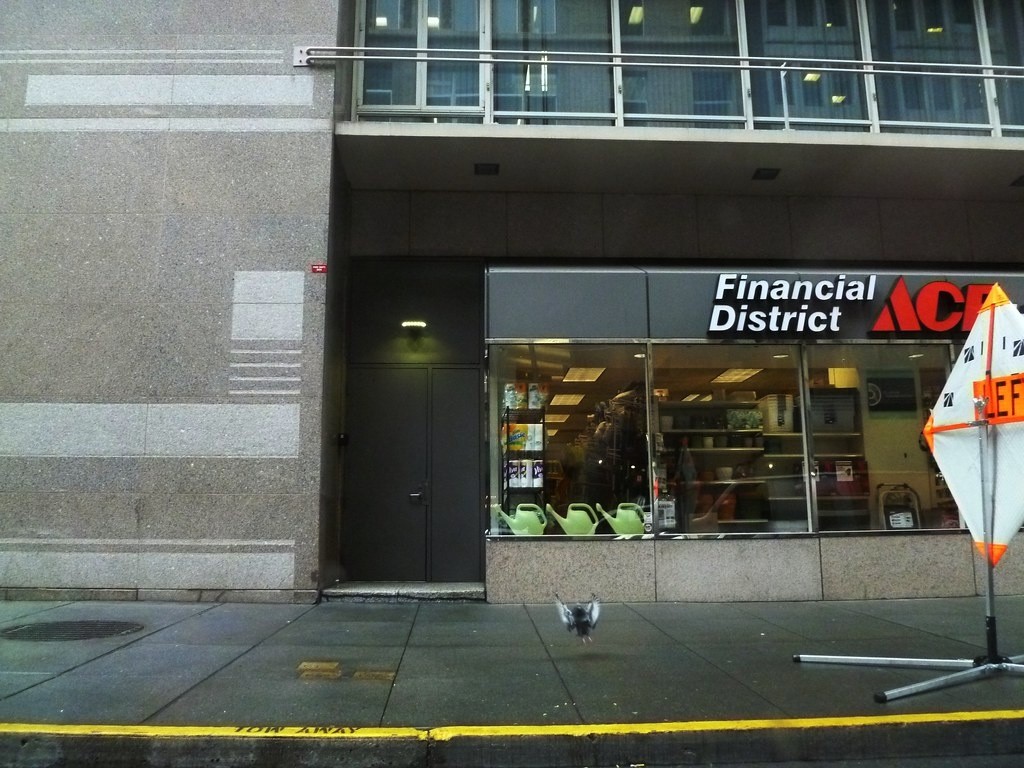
501, 406, 546, 533
763, 387, 874, 532
654, 388, 768, 524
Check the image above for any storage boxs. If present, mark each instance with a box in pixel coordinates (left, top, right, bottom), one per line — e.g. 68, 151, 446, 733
756, 394, 793, 433
798, 394, 854, 432
885, 504, 918, 529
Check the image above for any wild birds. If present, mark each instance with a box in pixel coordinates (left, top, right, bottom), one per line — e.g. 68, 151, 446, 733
553, 592, 604, 645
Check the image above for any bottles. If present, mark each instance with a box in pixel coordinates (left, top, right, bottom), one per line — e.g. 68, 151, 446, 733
672, 438, 697, 534
658, 489, 677, 530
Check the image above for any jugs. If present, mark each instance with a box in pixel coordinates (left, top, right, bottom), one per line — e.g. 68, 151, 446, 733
546, 499, 599, 534
494, 503, 546, 535
595, 502, 648, 533
